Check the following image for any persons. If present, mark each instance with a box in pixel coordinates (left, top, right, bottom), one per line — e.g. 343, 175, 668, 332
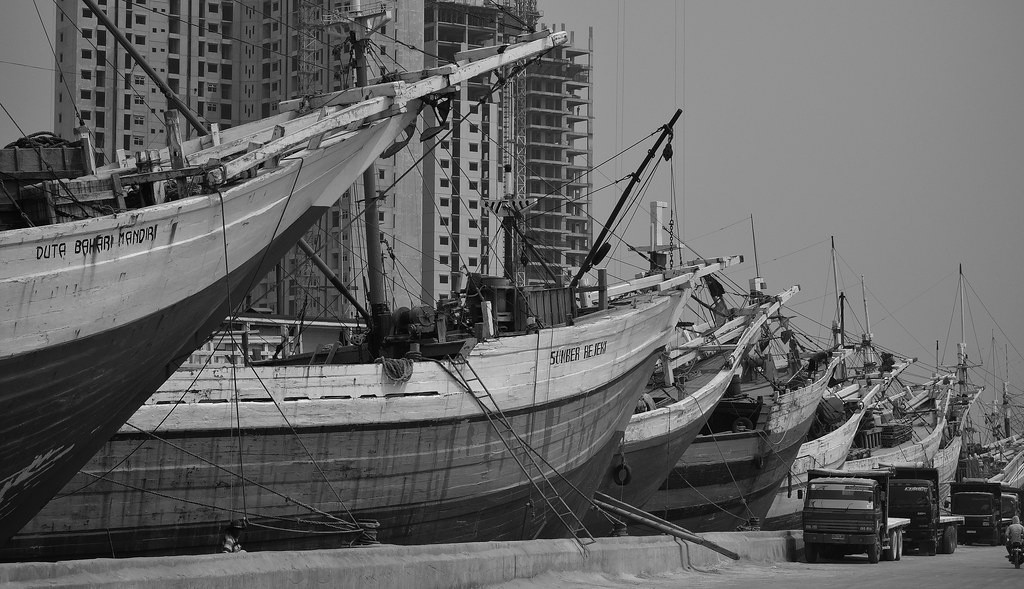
576, 105, 581, 115
1004, 516, 1024, 558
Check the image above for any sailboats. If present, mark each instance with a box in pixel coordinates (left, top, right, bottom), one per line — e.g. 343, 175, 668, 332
0, 0, 571, 544
588, 196, 1024, 529
12, 107, 744, 542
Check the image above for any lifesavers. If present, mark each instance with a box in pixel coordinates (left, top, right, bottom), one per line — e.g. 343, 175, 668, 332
612, 465, 634, 486
733, 419, 751, 433
593, 241, 611, 265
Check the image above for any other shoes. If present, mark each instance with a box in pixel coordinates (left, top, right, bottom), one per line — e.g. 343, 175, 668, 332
1006, 555, 1012, 558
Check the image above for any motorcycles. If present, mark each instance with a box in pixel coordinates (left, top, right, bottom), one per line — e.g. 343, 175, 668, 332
1006, 538, 1024, 569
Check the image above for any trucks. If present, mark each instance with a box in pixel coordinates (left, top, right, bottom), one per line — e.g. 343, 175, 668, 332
802, 467, 910, 564
950, 478, 1024, 546
873, 464, 965, 559
